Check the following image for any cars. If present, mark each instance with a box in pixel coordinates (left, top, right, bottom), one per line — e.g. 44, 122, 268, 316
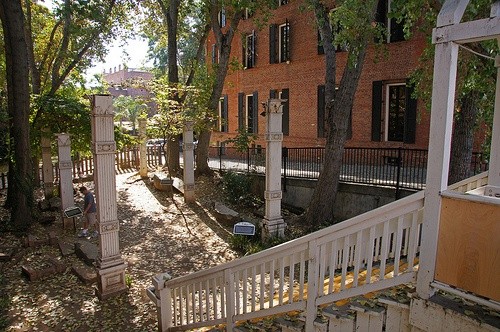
146, 139, 168, 153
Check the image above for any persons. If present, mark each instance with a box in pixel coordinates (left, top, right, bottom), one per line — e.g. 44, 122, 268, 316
78, 186, 99, 237
